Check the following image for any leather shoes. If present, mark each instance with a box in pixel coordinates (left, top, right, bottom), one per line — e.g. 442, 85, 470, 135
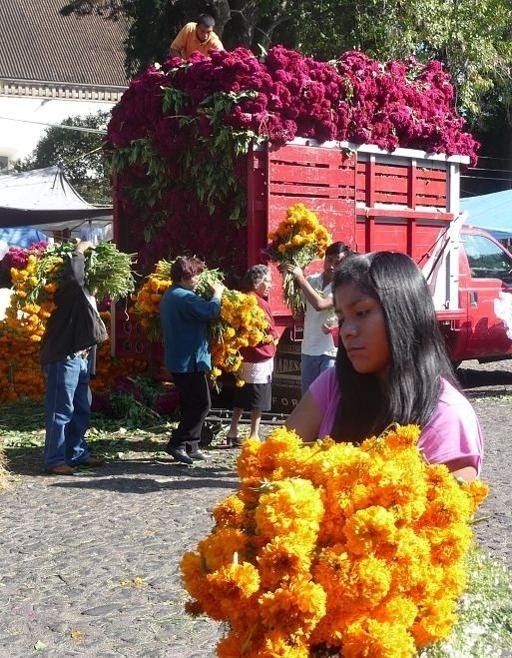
165, 445, 193, 463
188, 449, 209, 458
82, 453, 102, 466
53, 463, 74, 474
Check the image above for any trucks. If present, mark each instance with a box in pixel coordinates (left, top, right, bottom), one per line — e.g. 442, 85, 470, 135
109, 132, 511, 413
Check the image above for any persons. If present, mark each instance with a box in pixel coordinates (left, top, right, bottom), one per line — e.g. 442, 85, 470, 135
170, 13, 225, 60
285, 250, 484, 484
227, 265, 277, 448
159, 256, 225, 464
278, 240, 357, 399
42, 238, 110, 474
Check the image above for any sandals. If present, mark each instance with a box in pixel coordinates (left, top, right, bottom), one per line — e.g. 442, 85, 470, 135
225, 431, 247, 447
